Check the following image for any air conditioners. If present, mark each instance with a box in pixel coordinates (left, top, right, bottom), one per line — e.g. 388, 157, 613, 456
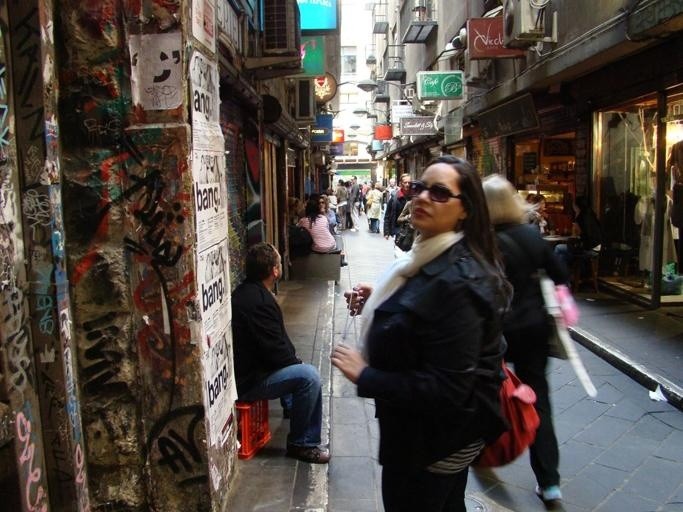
503, 0, 545, 50
264, 0, 301, 68
464, 49, 488, 84
295, 79, 316, 122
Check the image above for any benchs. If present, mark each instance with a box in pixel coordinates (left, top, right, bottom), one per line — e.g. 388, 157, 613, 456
292, 235, 342, 286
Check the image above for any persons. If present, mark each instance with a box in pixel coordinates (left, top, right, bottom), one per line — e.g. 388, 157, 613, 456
330, 155, 514, 511
529, 196, 554, 234
483, 172, 576, 502
548, 195, 608, 287
232, 243, 332, 465
288, 173, 421, 263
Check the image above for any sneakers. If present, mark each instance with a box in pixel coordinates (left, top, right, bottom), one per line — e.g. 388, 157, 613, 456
535, 484, 563, 500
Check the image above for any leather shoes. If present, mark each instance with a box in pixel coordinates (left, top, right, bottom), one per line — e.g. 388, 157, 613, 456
287, 443, 330, 463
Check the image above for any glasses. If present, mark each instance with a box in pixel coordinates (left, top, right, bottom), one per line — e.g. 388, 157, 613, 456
408, 182, 461, 202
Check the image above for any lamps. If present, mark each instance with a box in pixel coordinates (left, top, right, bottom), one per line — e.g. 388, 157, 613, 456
352, 107, 392, 126
358, 79, 416, 106
347, 133, 375, 138
349, 125, 374, 137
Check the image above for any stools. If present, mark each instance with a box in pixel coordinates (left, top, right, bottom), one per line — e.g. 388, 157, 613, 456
235, 401, 272, 461
572, 254, 600, 295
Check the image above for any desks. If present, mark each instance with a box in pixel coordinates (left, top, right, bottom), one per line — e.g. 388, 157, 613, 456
541, 235, 580, 251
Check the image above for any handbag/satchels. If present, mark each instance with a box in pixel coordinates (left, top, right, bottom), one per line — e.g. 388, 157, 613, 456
472, 359, 540, 468
395, 220, 415, 251
539, 277, 573, 359
366, 198, 373, 206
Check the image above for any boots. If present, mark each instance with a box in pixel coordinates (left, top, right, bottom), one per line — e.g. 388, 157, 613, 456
341, 255, 346, 266
